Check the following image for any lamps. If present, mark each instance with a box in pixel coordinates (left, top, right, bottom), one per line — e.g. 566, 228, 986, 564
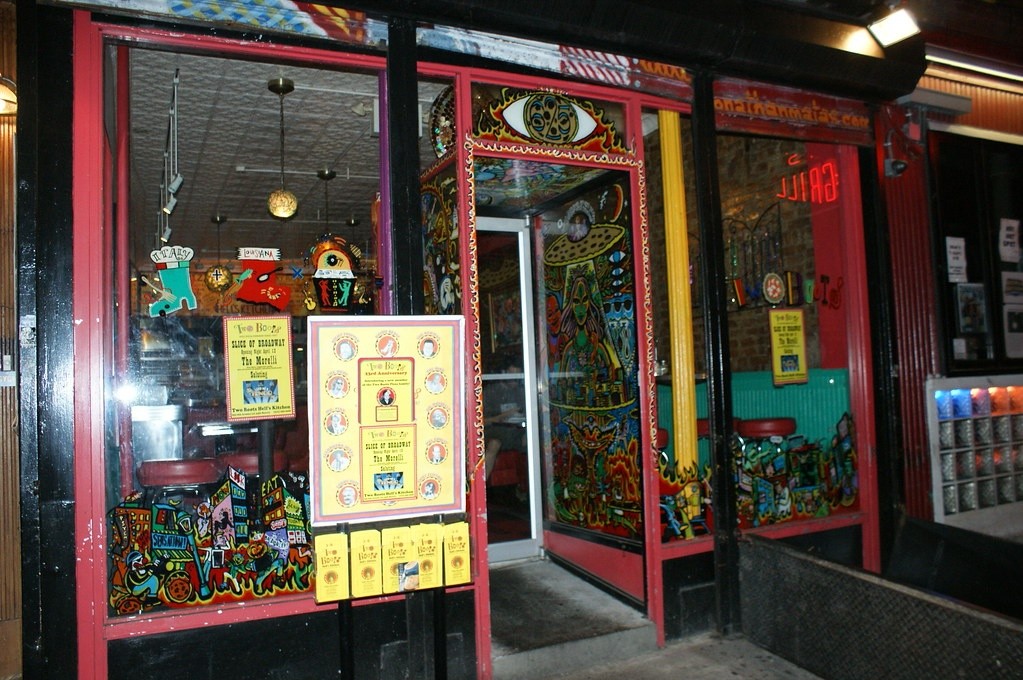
205, 216, 232, 292
864, 0, 923, 49
311, 169, 337, 268
161, 68, 183, 243
266, 78, 298, 219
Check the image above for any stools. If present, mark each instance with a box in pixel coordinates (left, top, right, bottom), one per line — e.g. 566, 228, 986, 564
136, 448, 289, 508
696, 417, 797, 455
